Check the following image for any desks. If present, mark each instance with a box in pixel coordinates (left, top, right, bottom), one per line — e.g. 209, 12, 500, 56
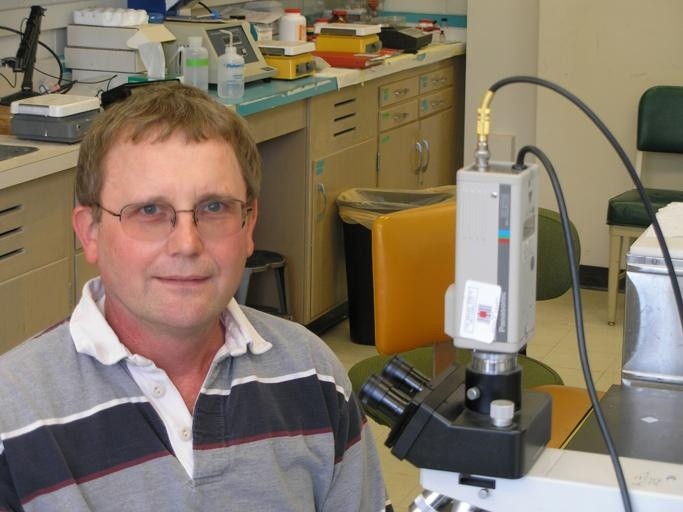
0, 37, 469, 364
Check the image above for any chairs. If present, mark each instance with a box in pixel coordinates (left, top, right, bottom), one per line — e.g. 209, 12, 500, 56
604, 85, 683, 327
373, 199, 605, 452
348, 209, 582, 455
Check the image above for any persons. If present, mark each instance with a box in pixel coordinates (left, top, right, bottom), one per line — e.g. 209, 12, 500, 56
0, 83, 386, 512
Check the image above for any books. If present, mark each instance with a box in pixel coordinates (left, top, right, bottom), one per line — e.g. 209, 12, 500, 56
312, 51, 385, 68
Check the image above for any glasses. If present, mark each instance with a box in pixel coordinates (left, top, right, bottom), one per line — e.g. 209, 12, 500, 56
91, 197, 254, 243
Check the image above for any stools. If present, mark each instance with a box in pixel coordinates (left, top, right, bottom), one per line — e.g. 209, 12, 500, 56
237, 248, 290, 322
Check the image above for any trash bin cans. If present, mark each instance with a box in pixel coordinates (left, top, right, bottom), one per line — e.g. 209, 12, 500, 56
336, 185, 456, 346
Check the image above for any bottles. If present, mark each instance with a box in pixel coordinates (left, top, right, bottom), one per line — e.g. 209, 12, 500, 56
279, 8, 306, 43
313, 18, 329, 34
182, 35, 209, 92
216, 29, 245, 98
418, 18, 449, 44
330, 9, 350, 25
